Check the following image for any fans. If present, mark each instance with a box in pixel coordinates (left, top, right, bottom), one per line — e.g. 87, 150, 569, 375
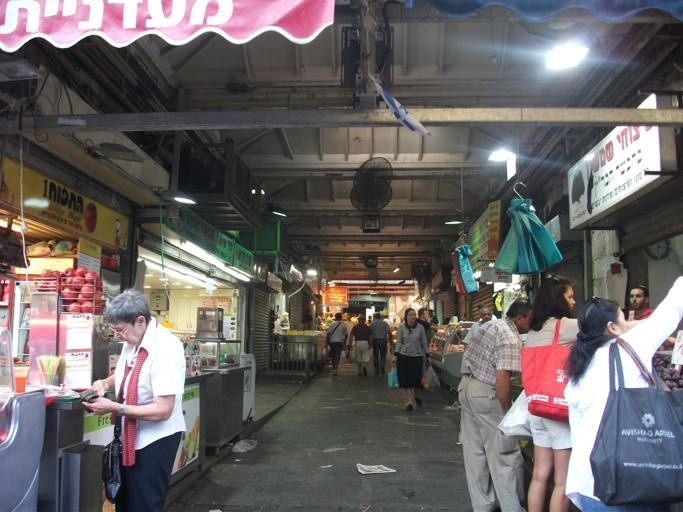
349, 158, 392, 214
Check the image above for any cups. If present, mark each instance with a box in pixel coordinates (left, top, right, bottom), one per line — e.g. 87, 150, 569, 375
14, 366, 28, 392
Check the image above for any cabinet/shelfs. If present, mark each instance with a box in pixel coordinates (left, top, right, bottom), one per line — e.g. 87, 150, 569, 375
428, 322, 477, 392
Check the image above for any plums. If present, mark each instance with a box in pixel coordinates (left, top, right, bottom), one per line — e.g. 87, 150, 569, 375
652, 356, 683, 391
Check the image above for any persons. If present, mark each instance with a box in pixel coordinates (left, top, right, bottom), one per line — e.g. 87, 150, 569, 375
81, 289, 187, 512
456, 275, 683, 512
391, 307, 431, 411
316, 309, 444, 378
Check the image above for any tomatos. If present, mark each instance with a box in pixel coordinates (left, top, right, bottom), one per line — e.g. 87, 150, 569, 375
85, 203, 97, 232
36, 267, 104, 314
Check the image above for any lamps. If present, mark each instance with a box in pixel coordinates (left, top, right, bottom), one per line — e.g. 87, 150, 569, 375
137, 240, 251, 291
156, 188, 198, 206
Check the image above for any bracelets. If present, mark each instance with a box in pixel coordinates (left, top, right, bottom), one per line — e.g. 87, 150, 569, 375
116, 404, 125, 417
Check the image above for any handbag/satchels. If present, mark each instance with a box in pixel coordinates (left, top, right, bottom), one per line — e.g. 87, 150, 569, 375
590, 388, 682, 507
99, 436, 127, 503
387, 366, 399, 389
519, 345, 573, 423
326, 333, 330, 343
